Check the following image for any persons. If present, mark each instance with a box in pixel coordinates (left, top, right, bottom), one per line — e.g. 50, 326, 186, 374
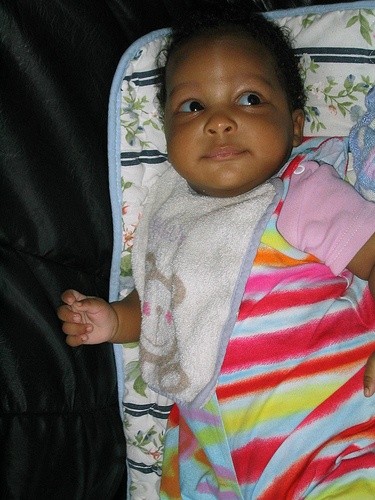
58, 2, 375, 500
